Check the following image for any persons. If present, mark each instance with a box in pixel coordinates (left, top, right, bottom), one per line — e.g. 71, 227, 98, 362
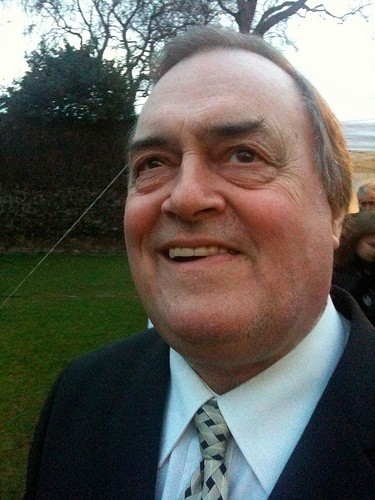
331, 210, 375, 326
357, 183, 375, 212
24, 25, 375, 500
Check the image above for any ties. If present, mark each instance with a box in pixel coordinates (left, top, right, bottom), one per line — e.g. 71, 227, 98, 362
182, 401, 232, 500
